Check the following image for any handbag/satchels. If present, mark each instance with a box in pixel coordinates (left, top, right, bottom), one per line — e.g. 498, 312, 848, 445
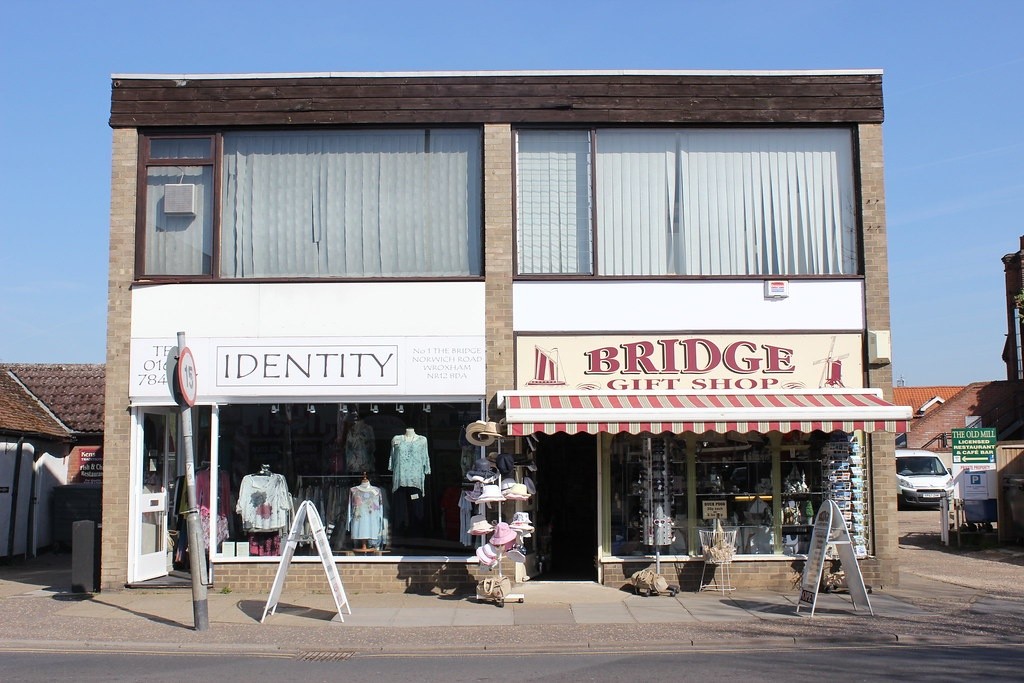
477, 577, 511, 598
631, 569, 669, 592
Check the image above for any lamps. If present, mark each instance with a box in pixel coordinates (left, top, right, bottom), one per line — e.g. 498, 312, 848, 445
422, 404, 432, 413
306, 404, 316, 414
271, 404, 280, 414
339, 404, 348, 414
370, 403, 380, 414
396, 404, 404, 413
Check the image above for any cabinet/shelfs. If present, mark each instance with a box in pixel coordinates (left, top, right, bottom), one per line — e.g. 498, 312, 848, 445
626, 459, 824, 553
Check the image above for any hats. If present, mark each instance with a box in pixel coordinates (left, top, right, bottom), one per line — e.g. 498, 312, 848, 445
465, 418, 537, 565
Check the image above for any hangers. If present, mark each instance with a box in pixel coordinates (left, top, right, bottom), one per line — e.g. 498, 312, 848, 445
303, 478, 356, 488
252, 464, 272, 477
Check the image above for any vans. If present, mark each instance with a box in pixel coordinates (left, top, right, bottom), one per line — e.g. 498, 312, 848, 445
895, 449, 954, 511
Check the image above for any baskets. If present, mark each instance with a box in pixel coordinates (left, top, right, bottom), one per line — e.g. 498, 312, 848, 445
700, 529, 736, 564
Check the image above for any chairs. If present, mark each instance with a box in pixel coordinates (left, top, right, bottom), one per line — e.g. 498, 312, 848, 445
699, 529, 737, 595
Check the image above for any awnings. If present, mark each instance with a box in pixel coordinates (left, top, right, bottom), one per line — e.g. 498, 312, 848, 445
497, 387, 916, 436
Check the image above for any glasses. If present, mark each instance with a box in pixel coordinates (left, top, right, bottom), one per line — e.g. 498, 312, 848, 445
632, 438, 676, 545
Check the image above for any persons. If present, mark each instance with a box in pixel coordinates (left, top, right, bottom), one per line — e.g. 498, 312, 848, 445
343, 420, 376, 474
388, 428, 431, 532
346, 479, 383, 540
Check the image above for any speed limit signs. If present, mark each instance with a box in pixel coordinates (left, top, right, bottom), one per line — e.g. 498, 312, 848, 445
178, 348, 197, 406
